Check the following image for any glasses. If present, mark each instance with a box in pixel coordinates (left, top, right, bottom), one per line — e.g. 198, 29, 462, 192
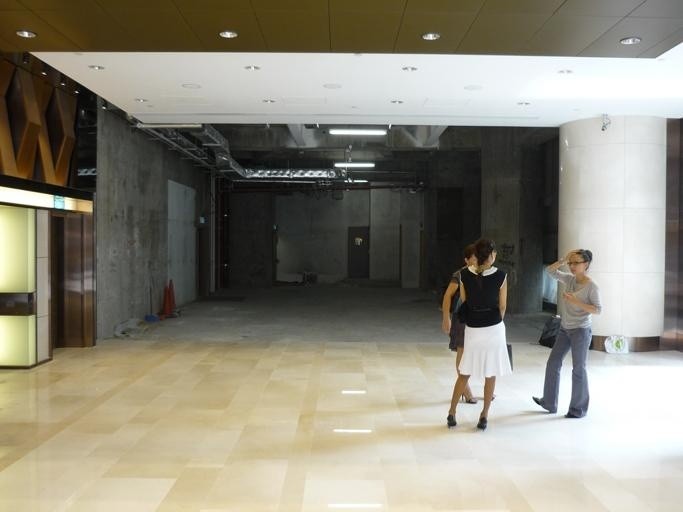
567, 261, 586, 266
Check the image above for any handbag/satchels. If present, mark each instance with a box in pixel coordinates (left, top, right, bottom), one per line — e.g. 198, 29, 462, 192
506, 344, 513, 370
438, 286, 461, 314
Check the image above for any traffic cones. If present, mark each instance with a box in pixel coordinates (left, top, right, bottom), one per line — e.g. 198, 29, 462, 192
167, 279, 177, 310
162, 285, 172, 315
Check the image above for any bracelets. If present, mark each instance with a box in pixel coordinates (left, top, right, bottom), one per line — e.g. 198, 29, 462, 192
558, 256, 568, 266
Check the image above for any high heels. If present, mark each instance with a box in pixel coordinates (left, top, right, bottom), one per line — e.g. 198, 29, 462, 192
461, 392, 477, 404
564, 413, 578, 418
477, 417, 487, 431
533, 397, 557, 413
447, 414, 457, 429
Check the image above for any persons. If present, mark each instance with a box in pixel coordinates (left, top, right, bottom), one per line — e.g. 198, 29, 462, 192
441, 243, 496, 404
446, 240, 506, 430
530, 248, 603, 417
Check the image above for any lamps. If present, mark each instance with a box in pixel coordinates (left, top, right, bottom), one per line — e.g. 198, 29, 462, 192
333, 160, 377, 170
327, 125, 389, 139
342, 179, 368, 186
